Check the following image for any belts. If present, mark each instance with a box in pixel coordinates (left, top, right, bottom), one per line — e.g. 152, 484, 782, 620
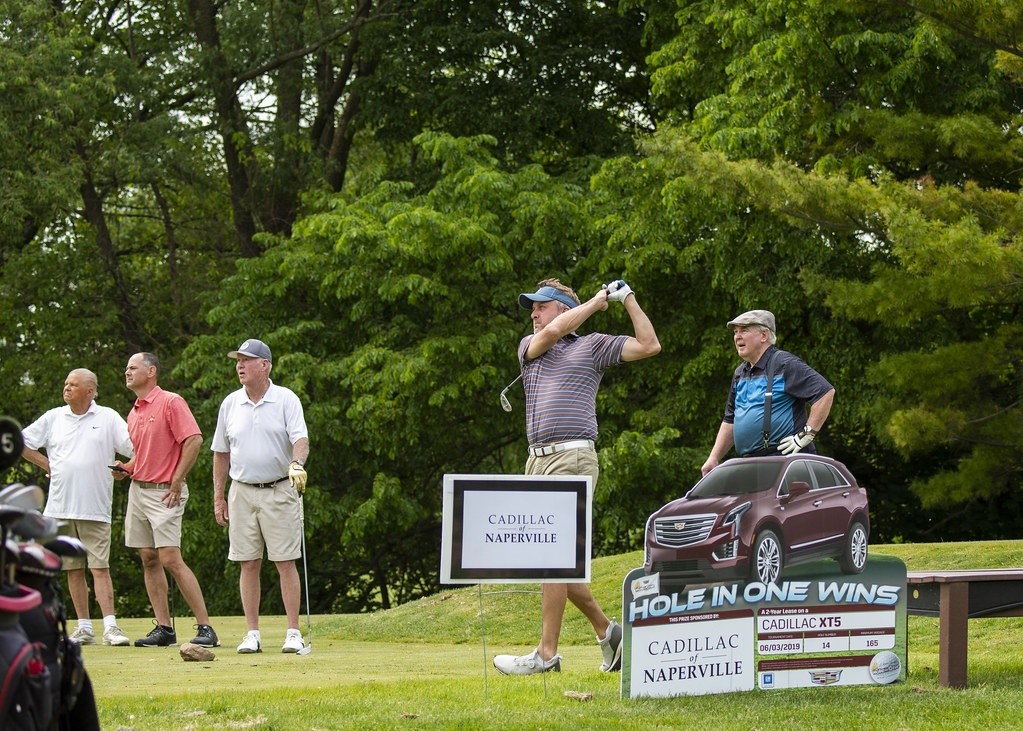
239, 476, 289, 488
140, 481, 170, 489
529, 440, 595, 457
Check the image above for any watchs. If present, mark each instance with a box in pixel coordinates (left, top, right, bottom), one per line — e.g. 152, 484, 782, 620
803, 424, 818, 434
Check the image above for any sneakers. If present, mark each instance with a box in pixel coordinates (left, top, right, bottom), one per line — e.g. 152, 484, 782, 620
492, 649, 563, 676
594, 616, 622, 674
189, 623, 221, 648
68, 626, 96, 645
134, 618, 177, 647
281, 633, 304, 652
236, 634, 262, 653
102, 625, 130, 646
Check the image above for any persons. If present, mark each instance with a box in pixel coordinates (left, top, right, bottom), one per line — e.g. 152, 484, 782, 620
493, 278, 661, 674
211, 339, 309, 653
701, 310, 835, 477
21, 368, 134, 646
111, 352, 221, 647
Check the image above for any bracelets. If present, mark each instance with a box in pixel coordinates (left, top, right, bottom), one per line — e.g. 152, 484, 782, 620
290, 458, 304, 466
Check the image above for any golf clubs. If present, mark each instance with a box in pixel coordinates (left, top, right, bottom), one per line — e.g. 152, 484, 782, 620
0, 481, 90, 586
499, 279, 625, 413
170, 574, 177, 643
295, 490, 313, 656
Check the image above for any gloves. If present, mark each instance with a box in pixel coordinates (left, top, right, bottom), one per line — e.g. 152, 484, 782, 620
777, 425, 815, 454
602, 280, 635, 305
288, 461, 308, 493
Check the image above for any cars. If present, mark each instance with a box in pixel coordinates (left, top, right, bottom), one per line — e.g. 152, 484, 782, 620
643, 453, 871, 586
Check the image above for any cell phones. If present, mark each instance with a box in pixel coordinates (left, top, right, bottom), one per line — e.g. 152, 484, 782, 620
108, 465, 130, 473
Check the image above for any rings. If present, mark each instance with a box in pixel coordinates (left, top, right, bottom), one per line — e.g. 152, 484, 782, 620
172, 497, 175, 499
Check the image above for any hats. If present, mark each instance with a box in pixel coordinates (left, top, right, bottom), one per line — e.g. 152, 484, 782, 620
726, 310, 775, 330
226, 339, 272, 363
518, 287, 576, 312
